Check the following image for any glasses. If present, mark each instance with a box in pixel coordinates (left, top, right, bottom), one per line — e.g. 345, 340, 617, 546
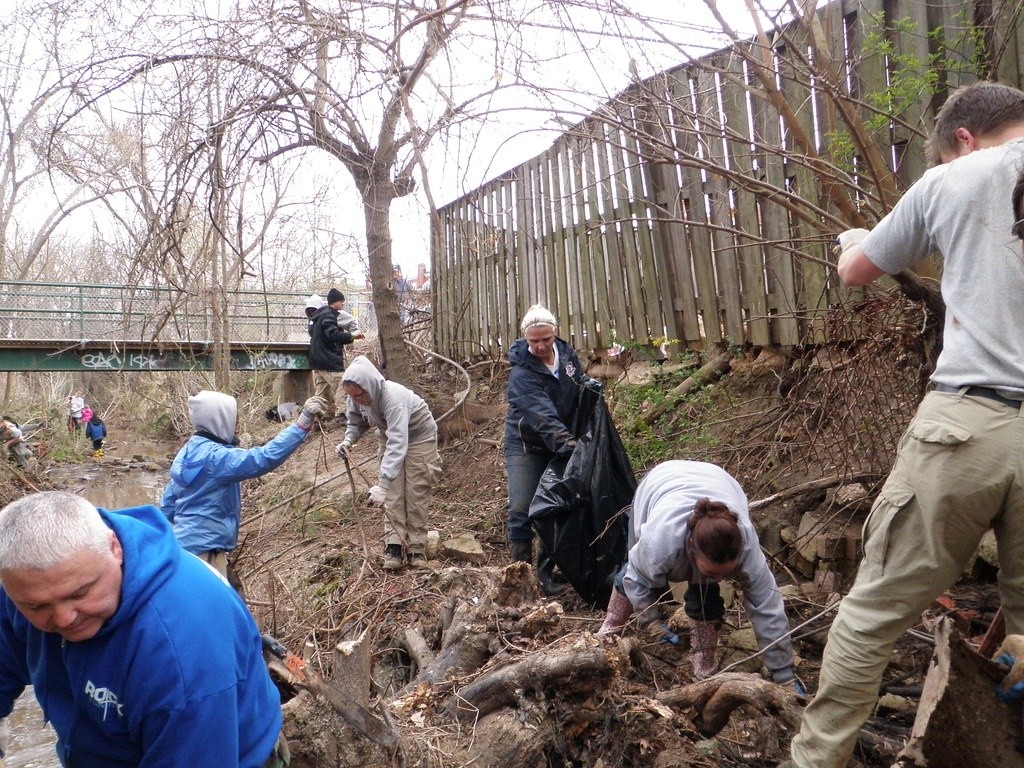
352, 392, 365, 401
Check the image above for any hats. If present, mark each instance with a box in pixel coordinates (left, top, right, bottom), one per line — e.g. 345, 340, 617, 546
266, 406, 278, 420
327, 288, 346, 306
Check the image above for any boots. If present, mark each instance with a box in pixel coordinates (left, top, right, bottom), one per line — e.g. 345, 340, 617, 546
597, 583, 636, 638
682, 603, 726, 682
509, 539, 533, 564
537, 540, 563, 596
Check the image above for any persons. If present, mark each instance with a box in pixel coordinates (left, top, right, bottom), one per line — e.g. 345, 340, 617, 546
308, 288, 365, 430
66, 390, 93, 439
992, 634, 1024, 700
0, 492, 290, 768
0, 416, 45, 458
790, 82, 1024, 768
598, 460, 802, 695
335, 356, 438, 569
305, 294, 358, 364
161, 391, 328, 578
268, 402, 299, 422
504, 304, 602, 593
86, 411, 107, 450
394, 266, 408, 326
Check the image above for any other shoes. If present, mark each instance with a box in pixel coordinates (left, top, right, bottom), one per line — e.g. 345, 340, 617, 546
335, 414, 348, 427
407, 553, 428, 568
314, 422, 327, 431
383, 545, 407, 570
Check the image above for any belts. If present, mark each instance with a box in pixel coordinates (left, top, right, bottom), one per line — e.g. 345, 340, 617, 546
934, 383, 1021, 409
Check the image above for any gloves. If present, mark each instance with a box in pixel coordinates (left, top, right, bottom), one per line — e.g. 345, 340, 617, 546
298, 396, 330, 429
366, 486, 388, 508
335, 441, 352, 463
782, 679, 803, 692
835, 228, 871, 250
647, 620, 678, 644
588, 378, 603, 389
989, 634, 1024, 701
